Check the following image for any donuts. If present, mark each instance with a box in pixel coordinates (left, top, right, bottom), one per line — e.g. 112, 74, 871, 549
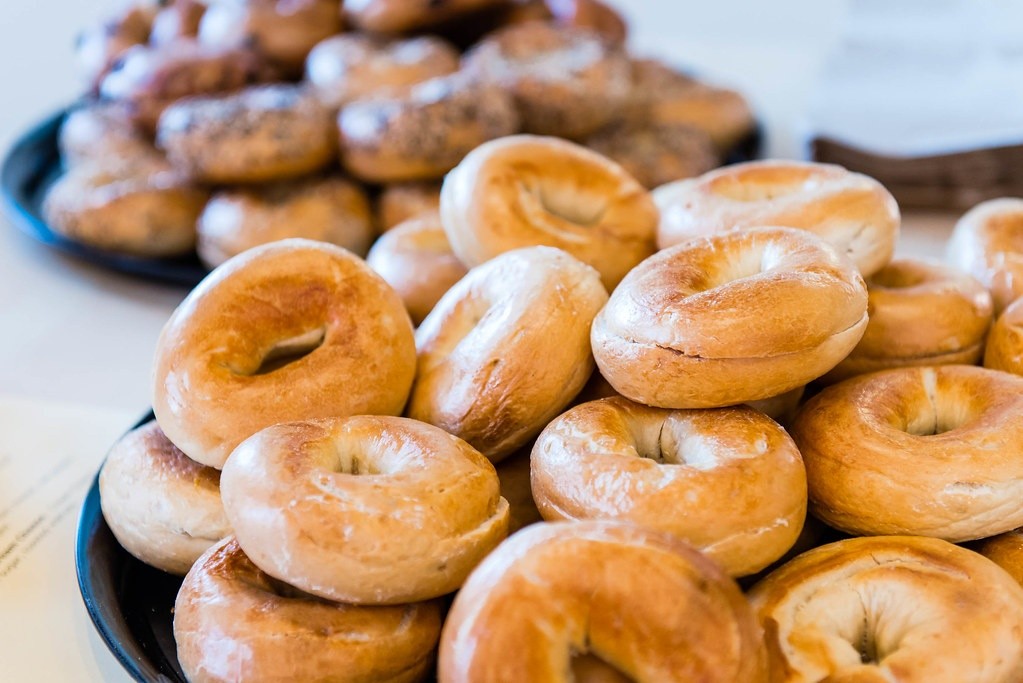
46, 0, 749, 277
94, 135, 1023, 683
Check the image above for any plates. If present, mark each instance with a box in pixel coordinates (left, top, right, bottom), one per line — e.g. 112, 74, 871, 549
0, 89, 765, 285
74, 383, 287, 682
